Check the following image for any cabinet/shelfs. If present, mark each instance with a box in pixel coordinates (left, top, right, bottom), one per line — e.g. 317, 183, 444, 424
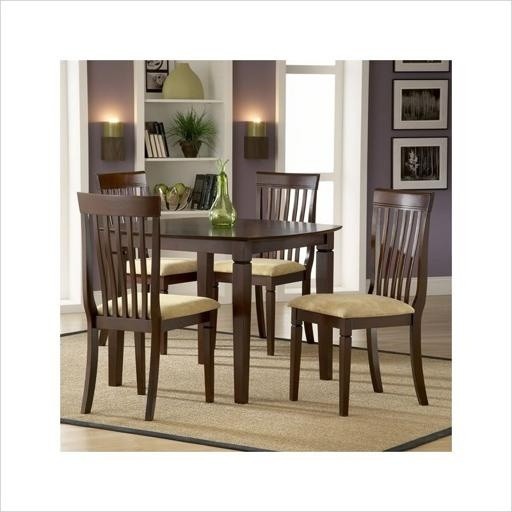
132, 60, 233, 218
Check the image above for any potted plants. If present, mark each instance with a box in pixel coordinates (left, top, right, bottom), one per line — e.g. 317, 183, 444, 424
208, 159, 237, 228
164, 104, 218, 158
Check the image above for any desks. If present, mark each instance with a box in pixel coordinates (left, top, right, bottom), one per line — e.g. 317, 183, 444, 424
97, 217, 343, 405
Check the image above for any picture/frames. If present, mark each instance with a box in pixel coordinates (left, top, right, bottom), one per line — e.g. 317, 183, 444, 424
145, 61, 170, 92
390, 60, 451, 191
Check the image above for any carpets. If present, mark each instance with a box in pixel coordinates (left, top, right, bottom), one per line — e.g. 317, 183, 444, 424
61, 326, 452, 451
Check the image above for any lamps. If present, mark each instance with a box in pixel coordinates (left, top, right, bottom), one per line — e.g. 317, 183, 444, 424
244, 121, 269, 159
101, 121, 126, 161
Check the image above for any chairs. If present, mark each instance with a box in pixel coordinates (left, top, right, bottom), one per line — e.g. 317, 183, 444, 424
96, 172, 197, 356
76, 192, 221, 424
289, 192, 433, 416
213, 173, 322, 357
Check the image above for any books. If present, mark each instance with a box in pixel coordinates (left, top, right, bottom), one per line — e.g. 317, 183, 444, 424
145, 121, 169, 158
191, 174, 218, 210
